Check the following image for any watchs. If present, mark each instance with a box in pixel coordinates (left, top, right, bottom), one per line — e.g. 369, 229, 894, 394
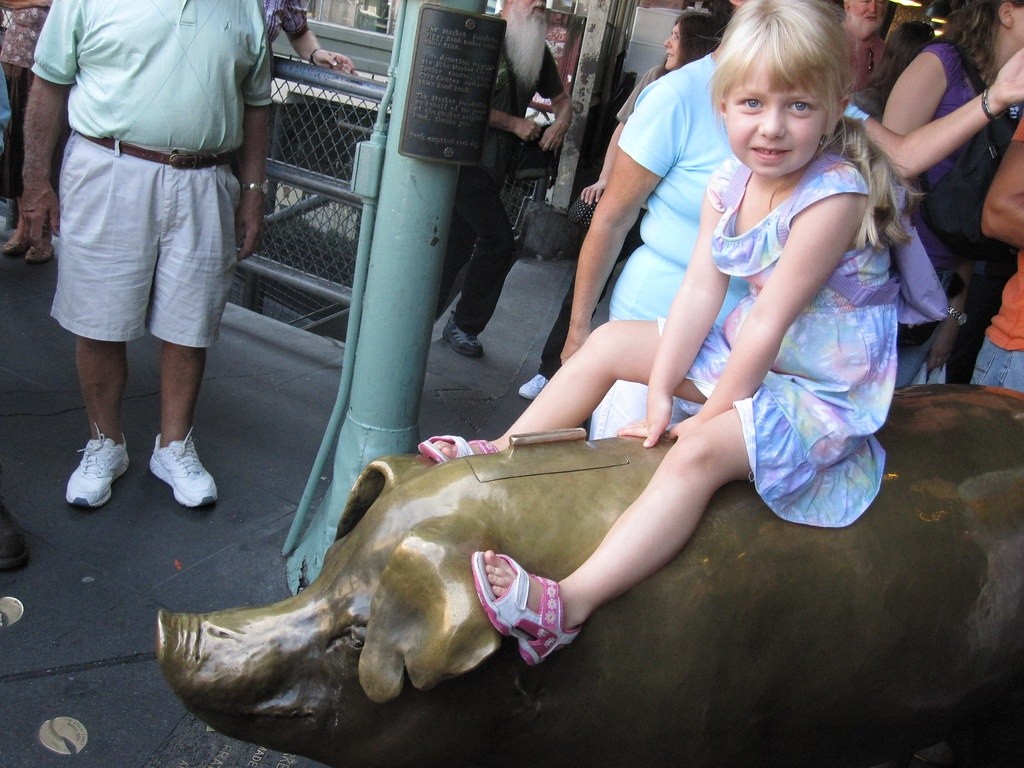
241, 178, 270, 196
949, 305, 968, 326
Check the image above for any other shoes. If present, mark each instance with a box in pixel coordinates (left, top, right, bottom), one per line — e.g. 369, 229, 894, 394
3, 241, 54, 263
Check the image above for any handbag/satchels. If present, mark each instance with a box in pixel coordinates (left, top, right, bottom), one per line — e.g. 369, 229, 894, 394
920, 119, 1010, 261
569, 195, 598, 229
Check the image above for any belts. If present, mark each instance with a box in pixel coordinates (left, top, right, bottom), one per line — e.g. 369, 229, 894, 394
76, 130, 225, 170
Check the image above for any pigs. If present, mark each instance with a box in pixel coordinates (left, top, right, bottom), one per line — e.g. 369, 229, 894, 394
155, 385, 1024, 768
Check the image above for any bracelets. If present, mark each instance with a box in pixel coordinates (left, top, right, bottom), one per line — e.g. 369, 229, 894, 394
982, 89, 1008, 120
310, 49, 317, 65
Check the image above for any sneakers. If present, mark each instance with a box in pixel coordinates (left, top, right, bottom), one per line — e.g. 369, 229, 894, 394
149, 433, 218, 507
443, 314, 483, 358
518, 373, 550, 399
67, 433, 129, 507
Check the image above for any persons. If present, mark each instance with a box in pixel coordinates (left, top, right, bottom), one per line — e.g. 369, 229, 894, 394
520, 12, 726, 401
842, 0, 1024, 392
20, 0, 273, 507
434, 0, 573, 357
264, 0, 358, 76
418, 1, 910, 666
0, 0, 69, 261
560, 0, 1024, 440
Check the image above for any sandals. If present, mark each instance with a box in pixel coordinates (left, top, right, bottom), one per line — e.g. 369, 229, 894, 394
471, 550, 581, 665
419, 436, 497, 463
